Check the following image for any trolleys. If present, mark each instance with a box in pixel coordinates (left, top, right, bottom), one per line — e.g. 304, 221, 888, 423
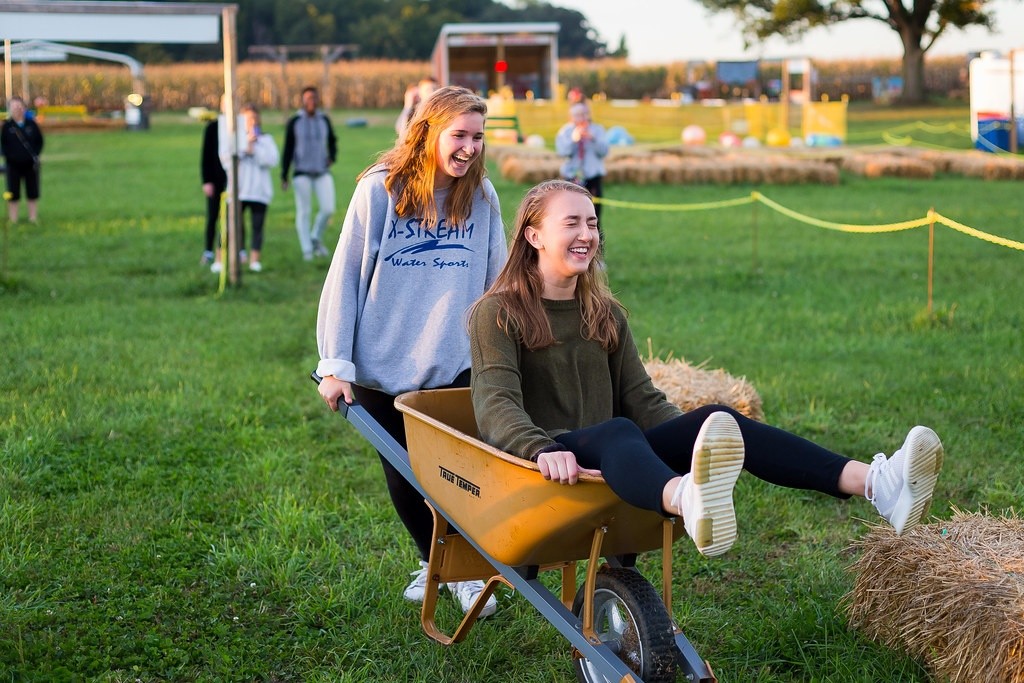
306, 368, 718, 682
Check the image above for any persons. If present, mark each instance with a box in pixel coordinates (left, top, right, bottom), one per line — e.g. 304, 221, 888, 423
281, 87, 337, 261
0, 96, 43, 226
315, 76, 508, 618
201, 92, 280, 273
557, 103, 610, 254
467, 175, 943, 559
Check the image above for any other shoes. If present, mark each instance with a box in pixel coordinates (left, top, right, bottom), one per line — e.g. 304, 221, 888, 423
314, 241, 328, 259
302, 252, 316, 263
8, 215, 18, 224
249, 258, 262, 272
202, 250, 214, 264
239, 249, 248, 265
29, 216, 40, 225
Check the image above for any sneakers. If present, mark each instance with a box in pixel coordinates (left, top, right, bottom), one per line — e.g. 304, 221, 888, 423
403, 560, 446, 606
446, 579, 497, 618
865, 425, 944, 538
671, 411, 745, 560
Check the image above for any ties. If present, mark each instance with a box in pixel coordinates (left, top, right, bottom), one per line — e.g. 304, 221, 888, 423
579, 139, 584, 161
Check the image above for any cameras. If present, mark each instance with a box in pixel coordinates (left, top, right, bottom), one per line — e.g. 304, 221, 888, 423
251, 125, 259, 137
